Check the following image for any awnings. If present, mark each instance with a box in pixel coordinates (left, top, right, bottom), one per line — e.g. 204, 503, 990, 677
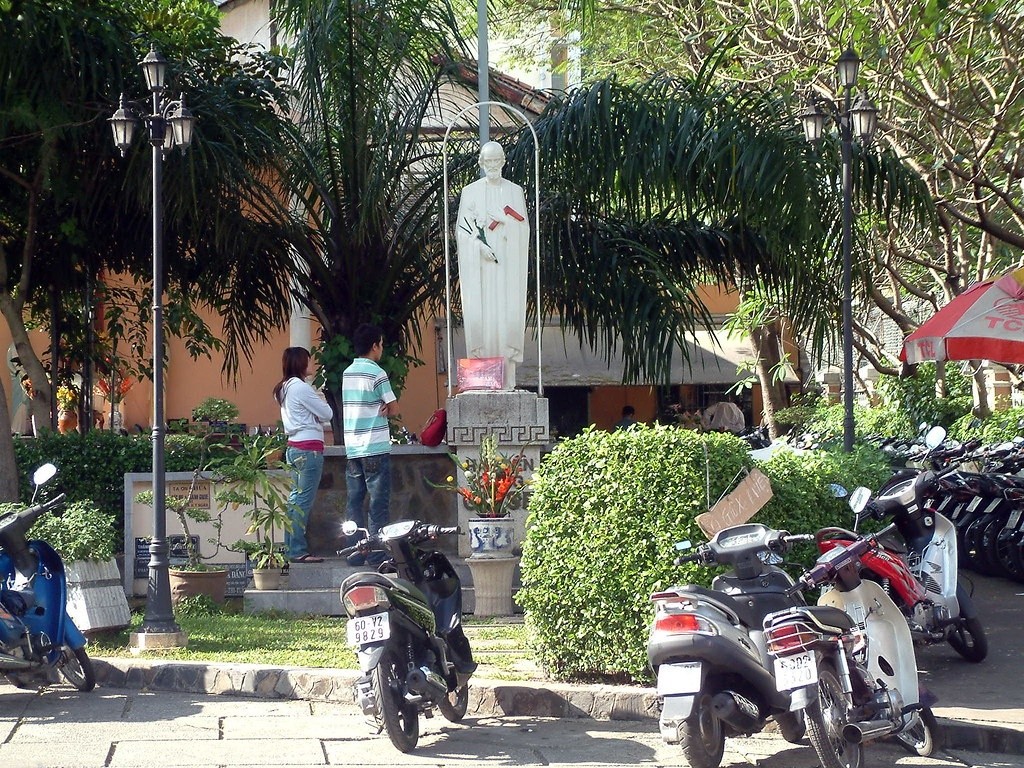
440, 327, 800, 387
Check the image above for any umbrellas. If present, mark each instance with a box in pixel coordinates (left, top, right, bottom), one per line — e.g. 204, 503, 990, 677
900, 267, 1024, 366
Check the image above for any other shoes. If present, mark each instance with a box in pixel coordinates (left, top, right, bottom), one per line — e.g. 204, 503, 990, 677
347, 557, 366, 566
368, 554, 395, 566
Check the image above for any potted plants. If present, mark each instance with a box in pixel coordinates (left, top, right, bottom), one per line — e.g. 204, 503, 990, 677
0, 498, 131, 633
134, 397, 258, 609
260, 420, 288, 469
202, 432, 307, 591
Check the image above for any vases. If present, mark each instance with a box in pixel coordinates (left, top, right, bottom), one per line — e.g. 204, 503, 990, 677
469, 513, 515, 559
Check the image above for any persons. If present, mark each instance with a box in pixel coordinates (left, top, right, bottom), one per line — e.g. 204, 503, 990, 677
702, 398, 745, 435
272, 346, 332, 564
458, 140, 528, 392
615, 406, 642, 431
343, 323, 400, 563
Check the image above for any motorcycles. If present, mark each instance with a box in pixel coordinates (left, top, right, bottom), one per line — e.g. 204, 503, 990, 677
763, 485, 943, 768
0, 462, 97, 693
645, 523, 811, 768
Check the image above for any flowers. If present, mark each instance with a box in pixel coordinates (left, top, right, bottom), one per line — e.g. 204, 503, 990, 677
422, 433, 536, 517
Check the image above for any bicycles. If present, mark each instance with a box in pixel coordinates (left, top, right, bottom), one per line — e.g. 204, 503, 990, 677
119, 419, 417, 447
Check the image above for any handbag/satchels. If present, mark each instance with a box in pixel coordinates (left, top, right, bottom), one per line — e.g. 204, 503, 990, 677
418, 408, 447, 448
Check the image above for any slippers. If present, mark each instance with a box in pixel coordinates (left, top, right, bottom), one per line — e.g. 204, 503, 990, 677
290, 554, 323, 563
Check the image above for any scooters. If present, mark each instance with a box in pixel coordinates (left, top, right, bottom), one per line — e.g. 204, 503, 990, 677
336, 517, 479, 753
733, 417, 1024, 583
814, 424, 989, 664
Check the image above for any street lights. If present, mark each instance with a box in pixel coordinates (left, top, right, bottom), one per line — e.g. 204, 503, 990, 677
796, 41, 880, 453
103, 41, 197, 632
